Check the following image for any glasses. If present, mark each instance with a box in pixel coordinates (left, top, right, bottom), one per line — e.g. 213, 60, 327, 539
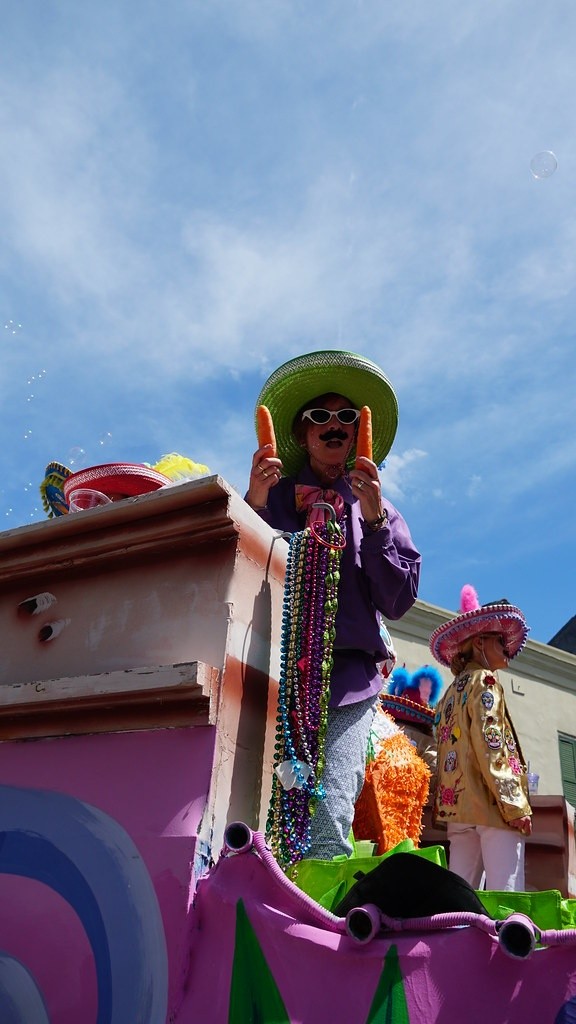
301, 408, 361, 425
479, 633, 505, 645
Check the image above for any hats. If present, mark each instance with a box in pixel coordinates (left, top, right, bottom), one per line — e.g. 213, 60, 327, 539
377, 662, 445, 728
254, 349, 401, 478
63, 451, 211, 509
37, 460, 74, 518
428, 584, 531, 668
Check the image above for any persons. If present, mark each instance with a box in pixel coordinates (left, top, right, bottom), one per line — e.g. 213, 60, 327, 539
245, 350, 421, 886
429, 583, 530, 893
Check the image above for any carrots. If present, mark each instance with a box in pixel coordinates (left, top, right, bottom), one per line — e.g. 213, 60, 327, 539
356, 406, 372, 475
257, 405, 278, 459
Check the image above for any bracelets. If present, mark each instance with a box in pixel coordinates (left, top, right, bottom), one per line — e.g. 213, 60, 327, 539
371, 509, 387, 526
309, 521, 347, 550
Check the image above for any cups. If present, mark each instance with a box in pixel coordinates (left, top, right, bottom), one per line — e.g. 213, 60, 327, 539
66, 488, 113, 514
526, 772, 540, 795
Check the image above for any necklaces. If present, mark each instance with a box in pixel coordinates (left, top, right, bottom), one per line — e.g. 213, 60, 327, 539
261, 523, 341, 886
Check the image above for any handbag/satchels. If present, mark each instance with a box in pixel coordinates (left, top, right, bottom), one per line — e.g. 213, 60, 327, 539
333, 851, 496, 921
473, 890, 576, 948
291, 835, 450, 912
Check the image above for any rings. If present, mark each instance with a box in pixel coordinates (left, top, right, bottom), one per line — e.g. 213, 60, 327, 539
258, 464, 263, 470
264, 472, 268, 476
357, 481, 364, 487
